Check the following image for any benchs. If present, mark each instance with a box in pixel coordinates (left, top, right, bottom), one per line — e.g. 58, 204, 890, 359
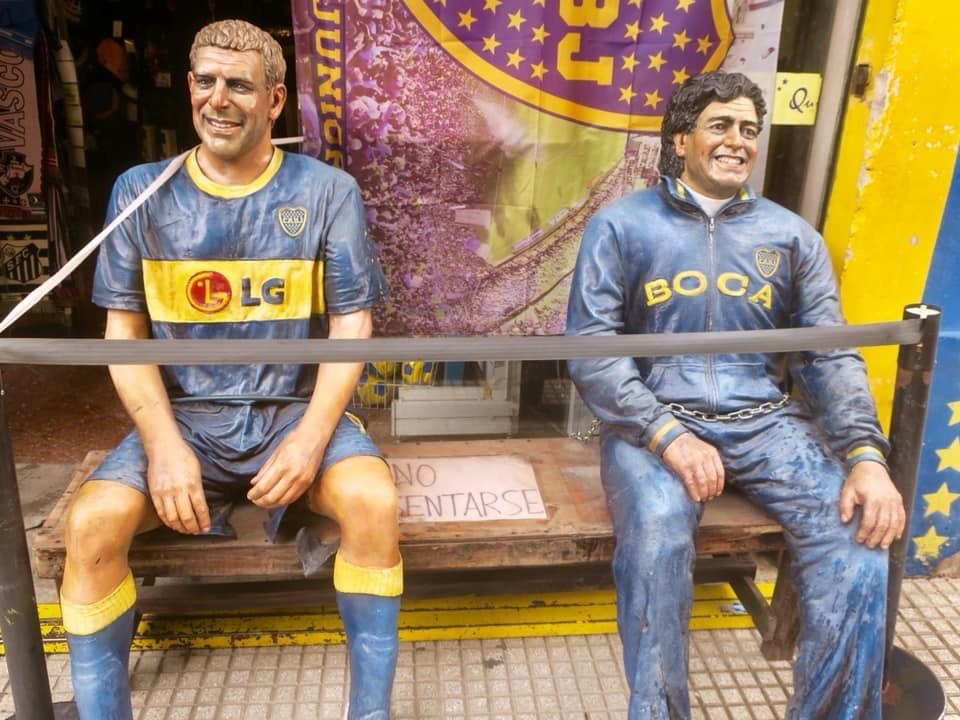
35, 434, 798, 661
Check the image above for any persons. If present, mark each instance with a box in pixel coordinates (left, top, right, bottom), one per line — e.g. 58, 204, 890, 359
562, 64, 912, 720
51, 16, 409, 720
